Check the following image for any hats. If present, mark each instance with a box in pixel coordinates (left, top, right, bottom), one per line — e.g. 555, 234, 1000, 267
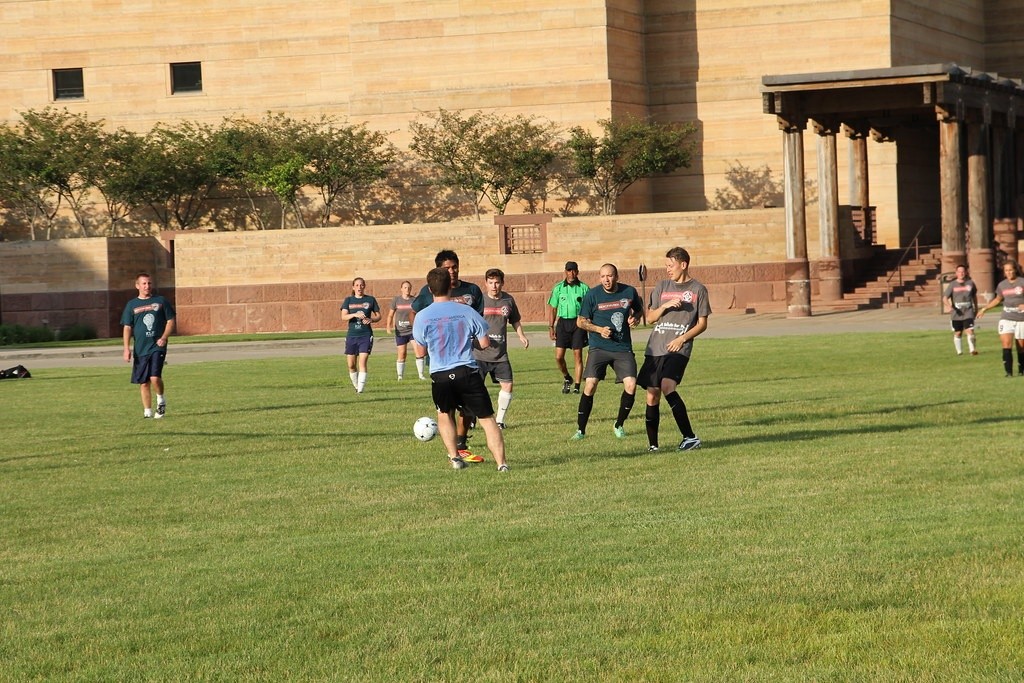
566, 262, 578, 270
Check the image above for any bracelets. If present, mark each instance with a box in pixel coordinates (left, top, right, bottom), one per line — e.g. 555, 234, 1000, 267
548, 325, 554, 329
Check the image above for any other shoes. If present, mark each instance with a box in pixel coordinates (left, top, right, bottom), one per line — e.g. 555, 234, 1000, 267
497, 422, 505, 431
970, 351, 976, 355
453, 458, 464, 469
420, 376, 427, 381
499, 466, 509, 473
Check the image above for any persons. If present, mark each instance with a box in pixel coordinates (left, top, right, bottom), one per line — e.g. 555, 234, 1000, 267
387, 280, 425, 381
943, 265, 978, 355
121, 273, 175, 418
412, 268, 508, 470
570, 264, 643, 439
635, 246, 712, 452
411, 250, 485, 462
977, 260, 1024, 377
547, 261, 590, 395
470, 268, 529, 429
340, 278, 382, 393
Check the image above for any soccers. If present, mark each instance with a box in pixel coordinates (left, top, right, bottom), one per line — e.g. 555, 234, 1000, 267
413, 417, 438, 441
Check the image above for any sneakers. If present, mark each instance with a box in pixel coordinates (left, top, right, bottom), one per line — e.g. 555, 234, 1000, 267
153, 399, 166, 419
447, 450, 484, 462
562, 376, 573, 394
676, 435, 701, 451
570, 431, 583, 441
613, 421, 624, 438
575, 389, 579, 394
647, 446, 657, 451
